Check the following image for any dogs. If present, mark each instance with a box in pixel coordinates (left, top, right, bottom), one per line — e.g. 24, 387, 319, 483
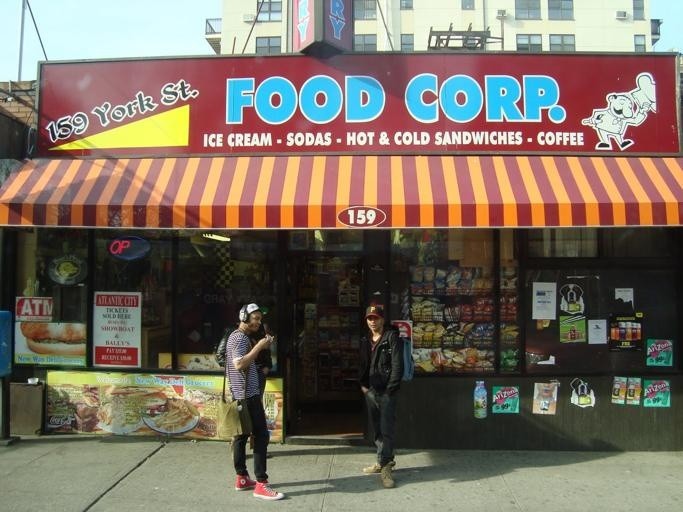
570, 377, 595, 408
561, 284, 584, 315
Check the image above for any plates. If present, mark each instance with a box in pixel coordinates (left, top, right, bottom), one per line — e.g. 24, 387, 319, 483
94, 398, 200, 434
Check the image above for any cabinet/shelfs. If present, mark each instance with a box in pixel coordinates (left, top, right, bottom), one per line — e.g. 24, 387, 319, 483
314, 314, 366, 400
409, 290, 524, 377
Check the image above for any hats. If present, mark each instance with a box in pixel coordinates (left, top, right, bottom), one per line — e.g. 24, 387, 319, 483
239, 303, 260, 322
366, 306, 382, 319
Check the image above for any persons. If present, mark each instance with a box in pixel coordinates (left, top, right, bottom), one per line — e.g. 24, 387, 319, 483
226, 303, 285, 500
215, 320, 272, 458
357, 306, 404, 488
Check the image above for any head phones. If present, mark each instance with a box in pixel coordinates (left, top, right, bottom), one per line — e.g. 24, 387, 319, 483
236, 304, 249, 323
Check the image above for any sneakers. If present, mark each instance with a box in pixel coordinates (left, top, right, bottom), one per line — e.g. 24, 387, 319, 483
236, 475, 256, 490
254, 479, 285, 500
363, 461, 396, 488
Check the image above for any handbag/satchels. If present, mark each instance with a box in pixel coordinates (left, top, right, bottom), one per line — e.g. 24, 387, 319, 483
216, 327, 237, 367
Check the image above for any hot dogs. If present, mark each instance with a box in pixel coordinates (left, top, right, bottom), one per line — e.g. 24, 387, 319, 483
21, 321, 87, 355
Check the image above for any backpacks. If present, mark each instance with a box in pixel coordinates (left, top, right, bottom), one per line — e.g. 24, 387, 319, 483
398, 335, 416, 382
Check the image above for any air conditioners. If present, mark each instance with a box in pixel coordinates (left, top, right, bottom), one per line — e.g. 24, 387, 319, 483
615, 10, 627, 19
495, 8, 507, 18
